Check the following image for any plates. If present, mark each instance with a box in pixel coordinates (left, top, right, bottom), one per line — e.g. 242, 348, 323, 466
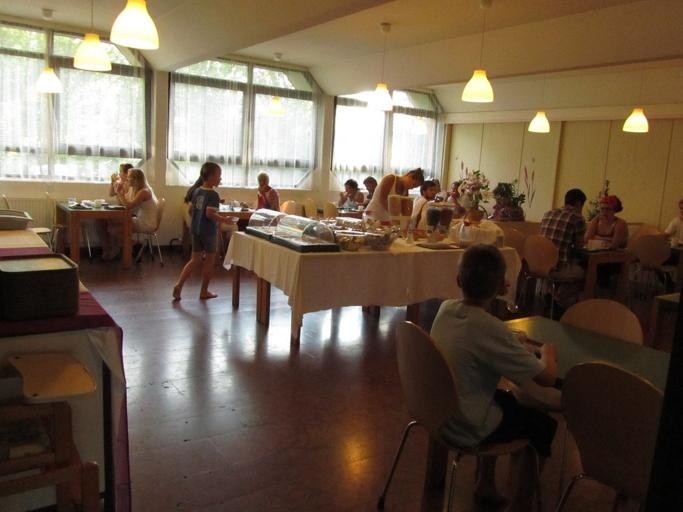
355, 210, 364, 212
101, 203, 124, 209
241, 208, 249, 211
415, 241, 450, 249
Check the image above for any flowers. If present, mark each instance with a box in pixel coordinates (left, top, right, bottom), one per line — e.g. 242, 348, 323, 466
456, 169, 490, 203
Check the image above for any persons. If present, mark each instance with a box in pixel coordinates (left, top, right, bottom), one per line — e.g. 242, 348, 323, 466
656, 199, 682, 295
363, 167, 426, 222
430, 243, 557, 512
488, 184, 527, 223
432, 179, 442, 193
535, 189, 587, 310
106, 168, 158, 253
171, 163, 235, 301
95, 163, 134, 261
181, 176, 240, 241
411, 181, 438, 218
338, 180, 365, 209
255, 173, 280, 226
362, 177, 379, 207
448, 182, 461, 205
584, 195, 628, 299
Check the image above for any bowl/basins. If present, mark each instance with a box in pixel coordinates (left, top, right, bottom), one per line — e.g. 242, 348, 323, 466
331, 214, 398, 251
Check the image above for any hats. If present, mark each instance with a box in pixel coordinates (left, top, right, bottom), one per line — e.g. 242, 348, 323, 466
600, 195, 622, 210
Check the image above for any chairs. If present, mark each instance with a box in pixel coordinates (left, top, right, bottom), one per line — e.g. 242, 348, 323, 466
1, 191, 166, 270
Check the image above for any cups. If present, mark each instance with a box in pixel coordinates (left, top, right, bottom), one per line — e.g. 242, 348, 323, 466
357, 205, 363, 211
68, 197, 105, 208
588, 239, 602, 251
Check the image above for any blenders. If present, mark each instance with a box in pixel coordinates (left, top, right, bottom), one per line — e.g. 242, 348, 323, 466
388, 194, 414, 238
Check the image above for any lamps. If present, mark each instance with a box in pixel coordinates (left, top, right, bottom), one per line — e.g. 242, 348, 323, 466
365, 1, 649, 134
31, 0, 161, 95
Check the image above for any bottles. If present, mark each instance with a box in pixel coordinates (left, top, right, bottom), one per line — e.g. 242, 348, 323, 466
335, 206, 352, 213
404, 224, 414, 244
426, 201, 455, 240
459, 222, 504, 249
